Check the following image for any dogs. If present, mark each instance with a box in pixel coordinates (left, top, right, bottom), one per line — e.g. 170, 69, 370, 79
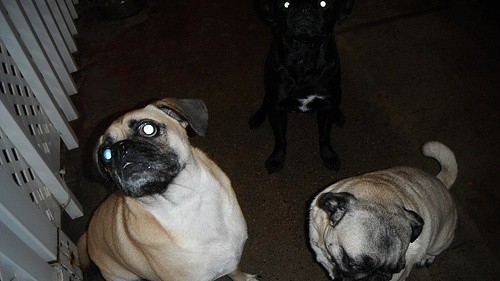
77, 97, 258, 281
248, 0, 355, 175
308, 141, 458, 280
